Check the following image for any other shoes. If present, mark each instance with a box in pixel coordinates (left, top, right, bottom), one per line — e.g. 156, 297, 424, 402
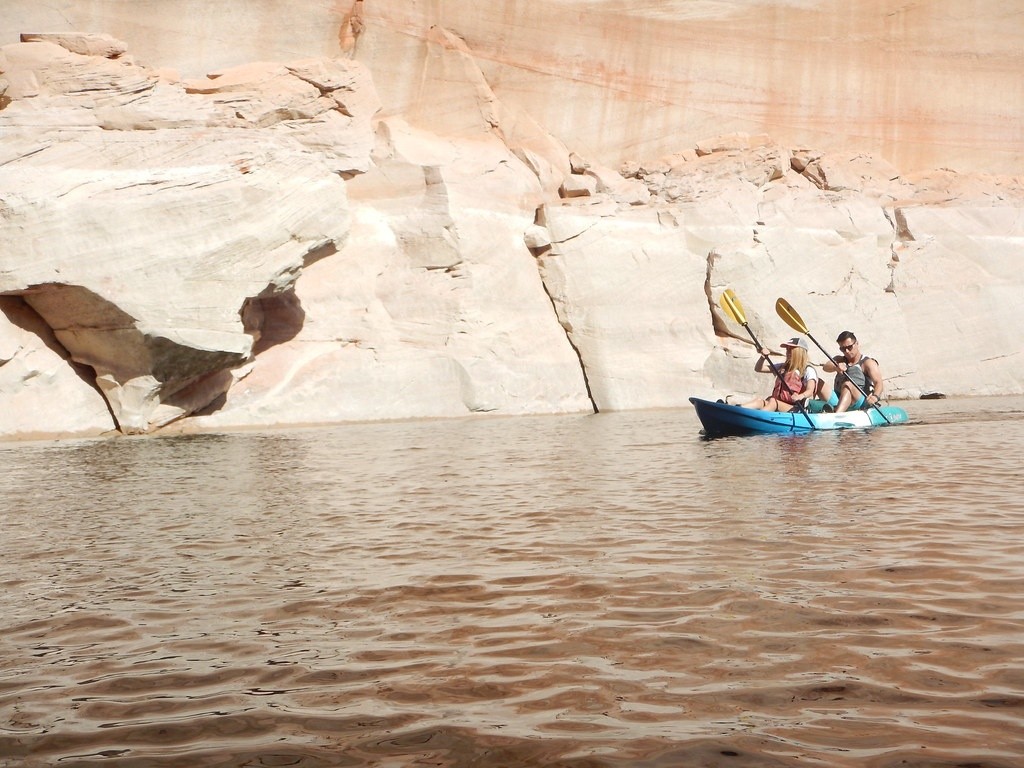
716, 398, 725, 403
822, 404, 834, 413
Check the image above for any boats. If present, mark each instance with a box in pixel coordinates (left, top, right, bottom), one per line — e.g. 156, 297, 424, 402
689, 396, 909, 437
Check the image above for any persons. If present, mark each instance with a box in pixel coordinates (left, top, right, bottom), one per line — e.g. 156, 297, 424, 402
812, 331, 882, 413
716, 338, 819, 413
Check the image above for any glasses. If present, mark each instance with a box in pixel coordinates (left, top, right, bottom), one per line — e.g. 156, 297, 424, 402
839, 341, 856, 352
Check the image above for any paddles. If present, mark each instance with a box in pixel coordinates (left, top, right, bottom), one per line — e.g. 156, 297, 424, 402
719, 288, 817, 430
775, 296, 892, 425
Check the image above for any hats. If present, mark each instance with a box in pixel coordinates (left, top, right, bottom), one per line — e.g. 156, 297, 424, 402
780, 338, 808, 351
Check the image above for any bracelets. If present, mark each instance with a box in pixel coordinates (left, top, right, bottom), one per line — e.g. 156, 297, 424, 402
873, 395, 879, 399
